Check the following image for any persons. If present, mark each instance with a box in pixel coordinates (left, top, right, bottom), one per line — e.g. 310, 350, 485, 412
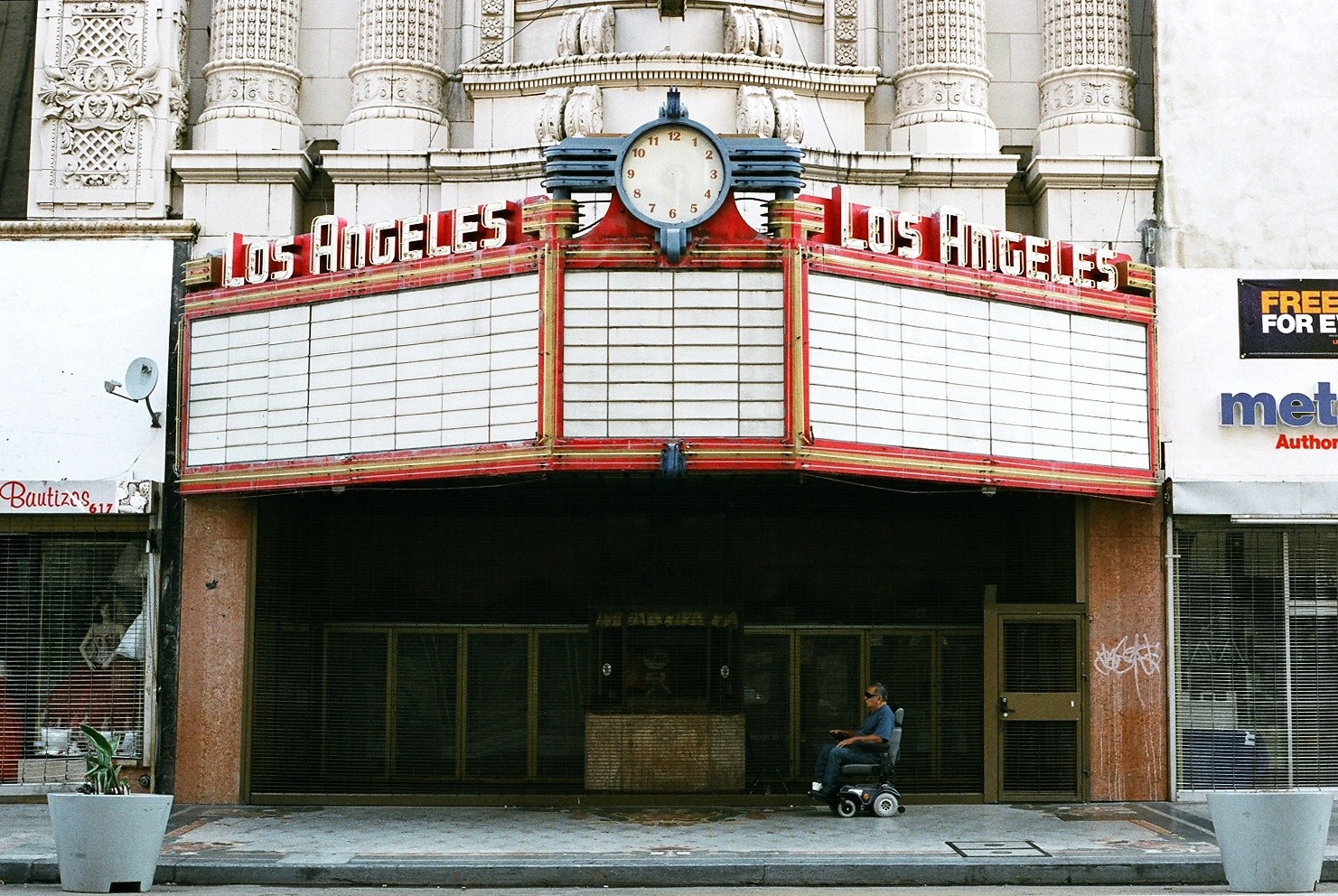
78, 601, 126, 730
808, 682, 895, 801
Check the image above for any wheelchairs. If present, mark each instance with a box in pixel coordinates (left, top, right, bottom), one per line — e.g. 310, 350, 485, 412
806, 708, 906, 817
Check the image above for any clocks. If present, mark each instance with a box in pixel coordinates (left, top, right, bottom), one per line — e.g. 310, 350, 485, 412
614, 118, 731, 228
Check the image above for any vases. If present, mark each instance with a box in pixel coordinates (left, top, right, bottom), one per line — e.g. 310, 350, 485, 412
1206, 792, 1333, 893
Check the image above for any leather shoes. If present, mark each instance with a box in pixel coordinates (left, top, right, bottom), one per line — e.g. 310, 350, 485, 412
805, 786, 835, 804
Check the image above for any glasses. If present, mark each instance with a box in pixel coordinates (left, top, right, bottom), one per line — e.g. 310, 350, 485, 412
864, 691, 877, 699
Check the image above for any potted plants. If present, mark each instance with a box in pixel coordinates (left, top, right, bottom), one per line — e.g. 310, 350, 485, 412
46, 723, 174, 892
